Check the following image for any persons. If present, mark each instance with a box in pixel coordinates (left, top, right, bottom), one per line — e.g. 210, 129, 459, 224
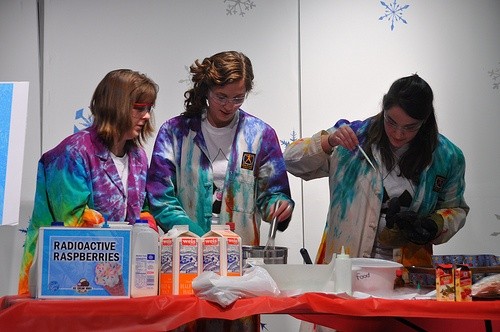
18, 69, 160, 296
283, 74, 469, 332
145, 50, 295, 332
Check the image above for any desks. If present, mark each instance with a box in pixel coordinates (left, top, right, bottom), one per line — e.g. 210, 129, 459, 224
0, 286, 500, 332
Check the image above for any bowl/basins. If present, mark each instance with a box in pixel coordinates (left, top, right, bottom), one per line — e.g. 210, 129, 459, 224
243, 244, 287, 266
348, 258, 403, 296
263, 264, 332, 293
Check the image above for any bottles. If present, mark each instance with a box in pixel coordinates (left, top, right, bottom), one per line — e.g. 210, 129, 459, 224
336, 245, 352, 297
131, 221, 159, 297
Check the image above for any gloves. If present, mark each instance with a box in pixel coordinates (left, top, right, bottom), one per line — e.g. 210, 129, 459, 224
378, 196, 445, 249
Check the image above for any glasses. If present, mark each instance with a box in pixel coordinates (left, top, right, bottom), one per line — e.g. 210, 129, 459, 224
384, 115, 424, 133
130, 103, 155, 118
208, 93, 250, 106
